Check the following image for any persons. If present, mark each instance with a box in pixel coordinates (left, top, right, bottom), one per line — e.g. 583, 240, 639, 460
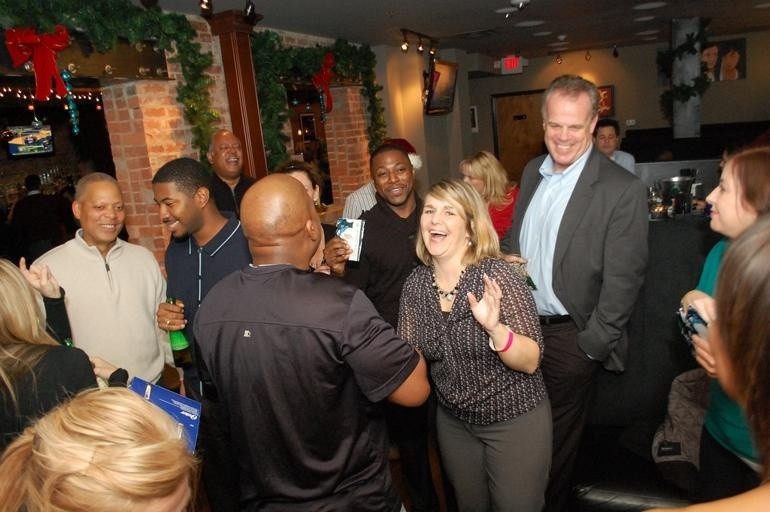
206, 130, 259, 221
692, 146, 744, 213
719, 45, 741, 82
342, 138, 422, 220
323, 145, 459, 512
397, 178, 553, 512
680, 147, 770, 505
193, 174, 429, 512
8, 174, 71, 268
700, 42, 718, 82
460, 151, 522, 242
0, 388, 193, 512
32, 172, 182, 395
0, 259, 127, 453
499, 73, 650, 512
643, 217, 770, 512
60, 185, 82, 242
153, 157, 253, 512
274, 159, 337, 276
591, 120, 635, 175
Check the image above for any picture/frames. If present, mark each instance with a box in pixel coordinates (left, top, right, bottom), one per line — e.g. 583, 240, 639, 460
470, 105, 478, 133
597, 85, 615, 118
701, 38, 747, 83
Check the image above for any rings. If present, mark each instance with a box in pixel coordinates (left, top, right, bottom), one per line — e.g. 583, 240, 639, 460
166, 319, 171, 327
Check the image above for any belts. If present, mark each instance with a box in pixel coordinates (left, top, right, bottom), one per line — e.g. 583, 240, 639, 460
537, 313, 575, 328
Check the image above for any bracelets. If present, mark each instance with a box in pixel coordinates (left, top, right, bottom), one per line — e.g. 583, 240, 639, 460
488, 325, 513, 353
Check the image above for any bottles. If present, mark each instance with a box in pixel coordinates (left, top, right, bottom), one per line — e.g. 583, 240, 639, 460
39, 167, 66, 191
167, 299, 192, 368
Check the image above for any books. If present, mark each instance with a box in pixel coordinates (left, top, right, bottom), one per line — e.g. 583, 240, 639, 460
336, 218, 366, 262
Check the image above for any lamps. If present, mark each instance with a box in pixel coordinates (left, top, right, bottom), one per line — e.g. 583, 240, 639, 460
585, 50, 591, 61
429, 40, 437, 55
555, 54, 564, 64
400, 32, 409, 51
612, 46, 619, 57
418, 37, 424, 52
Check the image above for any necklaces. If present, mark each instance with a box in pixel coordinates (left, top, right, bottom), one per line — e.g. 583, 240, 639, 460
428, 257, 471, 302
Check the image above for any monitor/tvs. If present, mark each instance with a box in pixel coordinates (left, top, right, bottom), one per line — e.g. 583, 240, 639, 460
425, 58, 458, 116
7, 122, 55, 160
